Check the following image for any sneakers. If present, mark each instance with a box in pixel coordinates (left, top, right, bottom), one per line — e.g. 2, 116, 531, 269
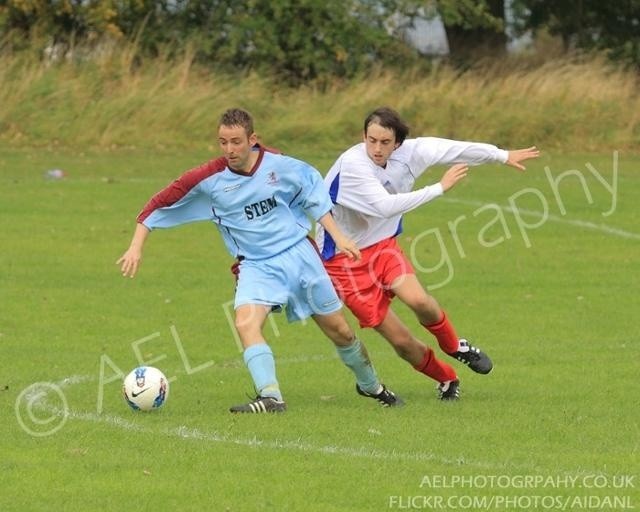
440, 339, 493, 374
437, 377, 460, 402
356, 383, 403, 406
230, 397, 286, 412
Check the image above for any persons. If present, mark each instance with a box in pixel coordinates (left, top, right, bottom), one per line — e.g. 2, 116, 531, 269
313, 106, 541, 400
114, 107, 408, 415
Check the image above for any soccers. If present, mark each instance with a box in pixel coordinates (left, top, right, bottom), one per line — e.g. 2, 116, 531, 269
123, 366, 169, 411
